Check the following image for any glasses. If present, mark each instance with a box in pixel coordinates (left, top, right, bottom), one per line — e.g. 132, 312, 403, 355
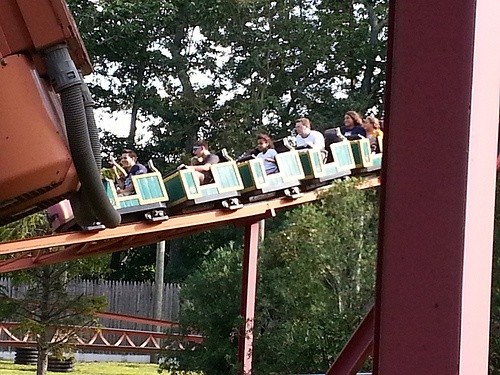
192, 146, 201, 151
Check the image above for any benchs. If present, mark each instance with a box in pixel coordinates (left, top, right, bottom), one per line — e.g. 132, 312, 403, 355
131, 120, 384, 195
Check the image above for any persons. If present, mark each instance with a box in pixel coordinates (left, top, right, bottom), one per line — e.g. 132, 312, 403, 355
362, 115, 384, 154
176, 139, 220, 185
291, 118, 325, 160
257, 134, 279, 175
104, 149, 147, 196
340, 111, 366, 140
